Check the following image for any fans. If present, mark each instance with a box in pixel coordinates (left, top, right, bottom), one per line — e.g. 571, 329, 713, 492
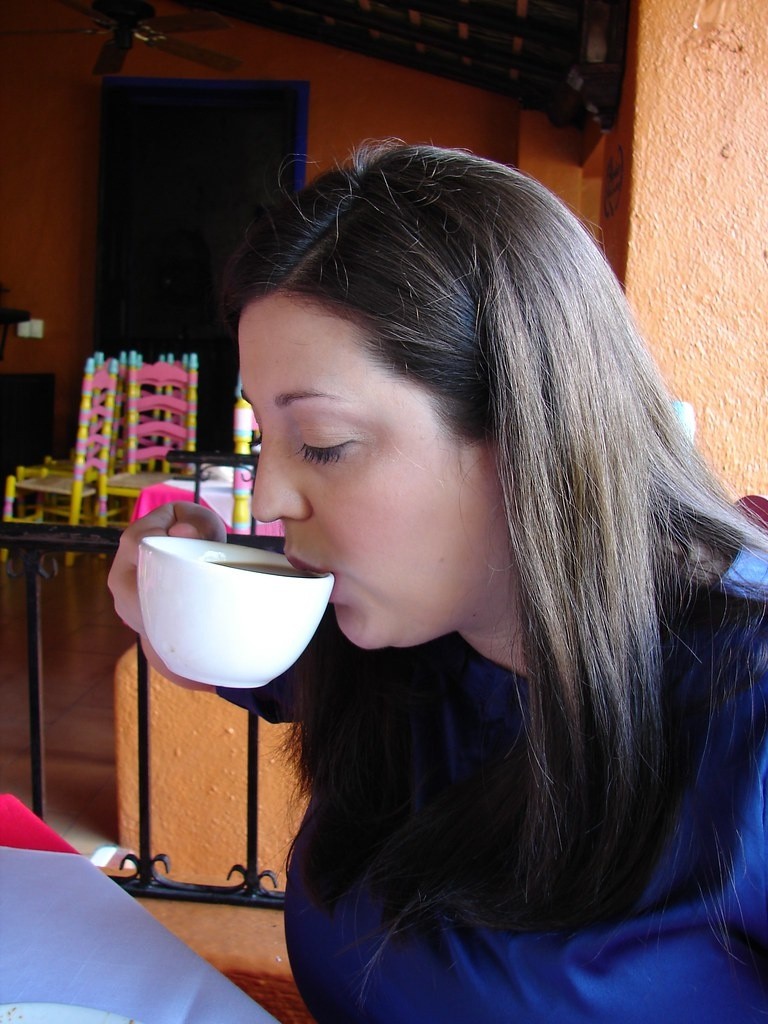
0, 0, 241, 77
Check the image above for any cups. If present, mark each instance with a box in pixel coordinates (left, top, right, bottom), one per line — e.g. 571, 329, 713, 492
138, 536, 335, 689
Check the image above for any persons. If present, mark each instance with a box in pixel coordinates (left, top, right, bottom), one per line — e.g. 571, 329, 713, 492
104, 139, 768, 1023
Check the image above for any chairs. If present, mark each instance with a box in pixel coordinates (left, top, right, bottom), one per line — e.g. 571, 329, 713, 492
4, 350, 198, 568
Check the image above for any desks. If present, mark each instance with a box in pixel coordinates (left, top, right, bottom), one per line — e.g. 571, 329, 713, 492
0, 791, 282, 1024
